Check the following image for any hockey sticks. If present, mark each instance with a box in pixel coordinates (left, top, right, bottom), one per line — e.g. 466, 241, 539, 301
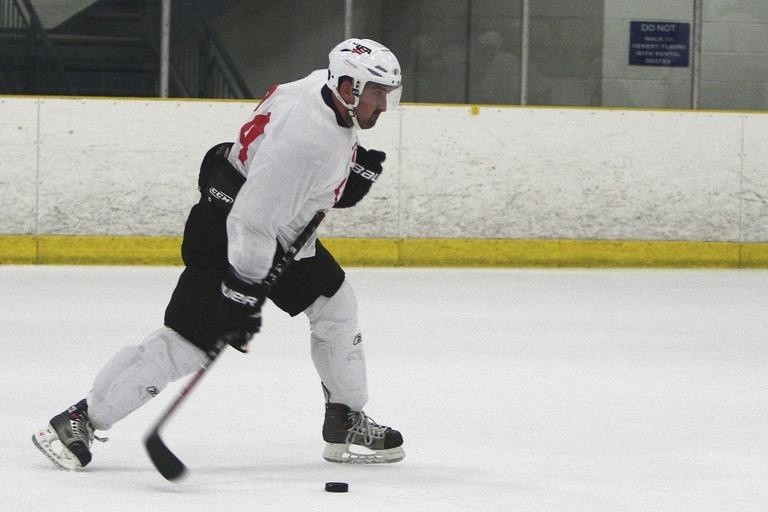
146, 210, 326, 480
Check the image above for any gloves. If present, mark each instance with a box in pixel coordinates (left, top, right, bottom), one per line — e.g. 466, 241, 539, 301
332, 145, 386, 208
200, 263, 267, 353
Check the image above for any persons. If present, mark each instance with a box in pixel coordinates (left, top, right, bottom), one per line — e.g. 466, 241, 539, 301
47, 37, 404, 470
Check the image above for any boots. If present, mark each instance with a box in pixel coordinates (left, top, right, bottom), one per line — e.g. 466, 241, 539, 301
49, 399, 92, 467
320, 381, 404, 451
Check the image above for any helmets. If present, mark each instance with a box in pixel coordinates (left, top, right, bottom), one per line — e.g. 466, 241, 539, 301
327, 38, 403, 109
